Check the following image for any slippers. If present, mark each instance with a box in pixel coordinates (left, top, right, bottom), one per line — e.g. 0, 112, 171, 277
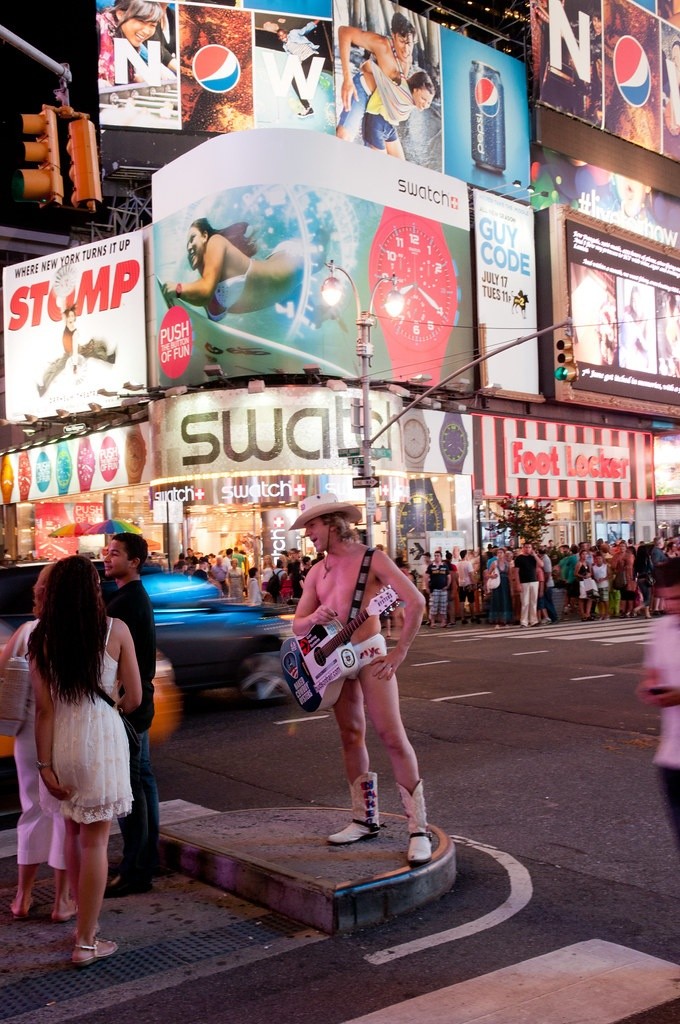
52, 899, 80, 923
10, 896, 36, 920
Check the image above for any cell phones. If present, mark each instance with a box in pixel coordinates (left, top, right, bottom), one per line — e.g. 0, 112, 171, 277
650, 688, 664, 696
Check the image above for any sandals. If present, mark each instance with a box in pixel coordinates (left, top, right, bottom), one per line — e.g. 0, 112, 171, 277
72, 938, 118, 967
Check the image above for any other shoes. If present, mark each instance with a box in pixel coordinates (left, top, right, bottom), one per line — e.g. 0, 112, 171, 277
430, 624, 437, 628
462, 620, 468, 625
472, 619, 477, 623
440, 624, 447, 628
104, 874, 152, 898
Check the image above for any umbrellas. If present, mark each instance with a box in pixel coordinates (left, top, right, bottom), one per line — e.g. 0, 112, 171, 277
47, 519, 141, 537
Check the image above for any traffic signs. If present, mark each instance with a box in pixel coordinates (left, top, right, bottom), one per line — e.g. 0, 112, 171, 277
352, 477, 380, 488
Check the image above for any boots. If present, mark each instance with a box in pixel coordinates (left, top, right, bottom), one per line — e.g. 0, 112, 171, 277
327, 771, 380, 844
394, 777, 432, 863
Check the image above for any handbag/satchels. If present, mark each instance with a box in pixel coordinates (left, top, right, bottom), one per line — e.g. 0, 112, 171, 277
628, 581, 638, 592
509, 567, 523, 594
578, 578, 599, 599
0, 620, 33, 738
488, 560, 501, 590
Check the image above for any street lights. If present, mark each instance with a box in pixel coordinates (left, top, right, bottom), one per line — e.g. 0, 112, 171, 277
321, 257, 405, 547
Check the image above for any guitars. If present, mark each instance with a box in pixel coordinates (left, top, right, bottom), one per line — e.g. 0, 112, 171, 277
279, 583, 401, 713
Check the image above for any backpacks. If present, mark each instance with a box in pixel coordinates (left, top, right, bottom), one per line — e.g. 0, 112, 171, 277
267, 569, 284, 592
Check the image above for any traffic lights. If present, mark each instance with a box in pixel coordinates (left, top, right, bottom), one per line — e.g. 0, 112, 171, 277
554, 338, 580, 383
65, 118, 102, 212
9, 108, 64, 208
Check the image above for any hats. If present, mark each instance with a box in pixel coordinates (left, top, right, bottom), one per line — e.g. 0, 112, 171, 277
289, 493, 363, 531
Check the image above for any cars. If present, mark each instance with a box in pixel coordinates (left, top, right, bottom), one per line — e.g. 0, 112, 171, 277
0, 556, 298, 709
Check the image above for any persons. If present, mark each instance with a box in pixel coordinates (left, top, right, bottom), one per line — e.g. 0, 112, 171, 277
96, 0, 213, 103
595, 284, 680, 376
158, 218, 296, 314
637, 571, 680, 849
104, 532, 160, 899
0, 564, 79, 921
27, 556, 141, 965
4, 549, 34, 561
582, 9, 628, 130
147, 547, 325, 604
288, 492, 431, 863
376, 537, 680, 637
278, 20, 319, 118
337, 14, 435, 160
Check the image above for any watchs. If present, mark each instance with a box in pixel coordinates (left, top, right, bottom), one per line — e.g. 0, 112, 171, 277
0, 425, 148, 504
176, 283, 182, 298
36, 760, 51, 768
396, 409, 467, 547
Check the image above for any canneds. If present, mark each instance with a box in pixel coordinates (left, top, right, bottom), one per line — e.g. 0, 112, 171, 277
468, 60, 506, 174
